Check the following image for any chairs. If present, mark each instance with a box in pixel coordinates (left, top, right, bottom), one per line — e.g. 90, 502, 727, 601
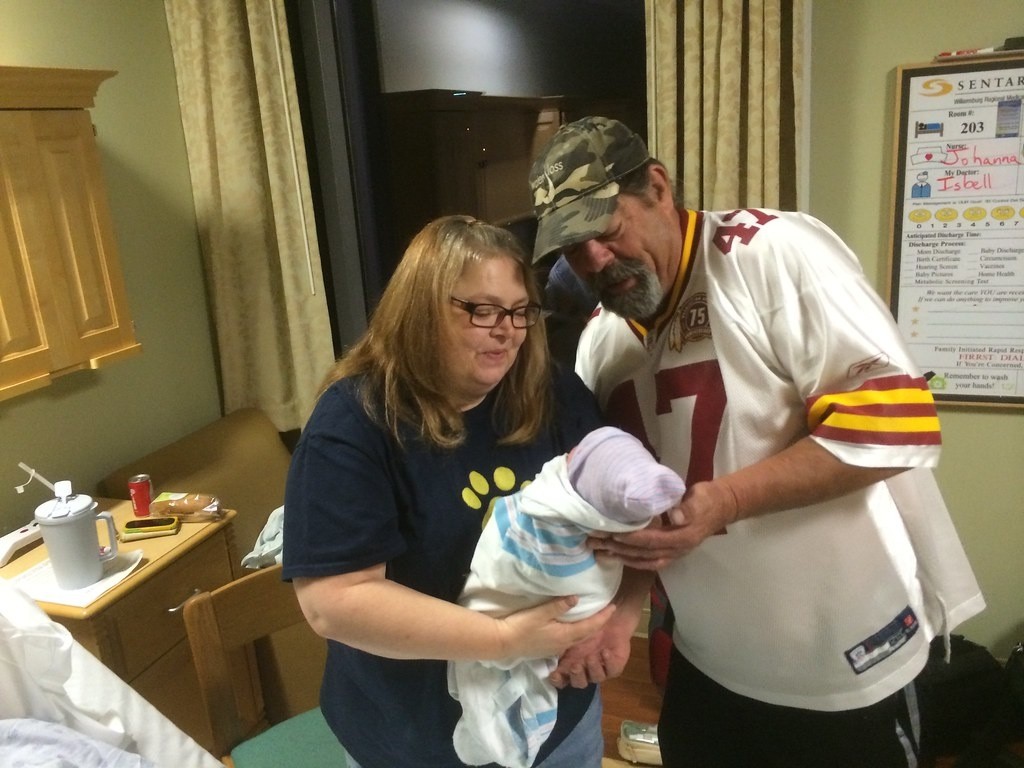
183, 563, 346, 768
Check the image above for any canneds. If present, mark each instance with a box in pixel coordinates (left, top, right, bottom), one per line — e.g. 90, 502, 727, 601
128, 474, 155, 516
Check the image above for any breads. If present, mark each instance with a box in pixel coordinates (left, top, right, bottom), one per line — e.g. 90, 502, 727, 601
150, 492, 215, 523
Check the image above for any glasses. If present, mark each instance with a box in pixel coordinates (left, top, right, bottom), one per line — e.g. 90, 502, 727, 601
449, 296, 541, 328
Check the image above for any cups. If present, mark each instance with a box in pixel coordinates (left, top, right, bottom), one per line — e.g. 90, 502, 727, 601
33, 494, 118, 590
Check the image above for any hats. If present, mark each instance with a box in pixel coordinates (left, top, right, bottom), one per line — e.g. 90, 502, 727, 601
567, 426, 687, 522
528, 116, 650, 266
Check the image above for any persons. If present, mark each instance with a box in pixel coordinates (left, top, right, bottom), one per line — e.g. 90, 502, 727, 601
279, 213, 645, 767
529, 116, 987, 768
444, 426, 685, 767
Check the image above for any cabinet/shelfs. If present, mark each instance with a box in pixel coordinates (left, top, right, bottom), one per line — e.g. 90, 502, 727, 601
0, 66, 142, 401
0, 495, 270, 768
378, 88, 648, 263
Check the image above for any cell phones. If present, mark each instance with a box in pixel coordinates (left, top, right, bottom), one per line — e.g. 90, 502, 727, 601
122, 516, 179, 533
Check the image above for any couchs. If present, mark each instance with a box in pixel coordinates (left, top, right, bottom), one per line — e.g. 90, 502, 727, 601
99, 407, 327, 725
